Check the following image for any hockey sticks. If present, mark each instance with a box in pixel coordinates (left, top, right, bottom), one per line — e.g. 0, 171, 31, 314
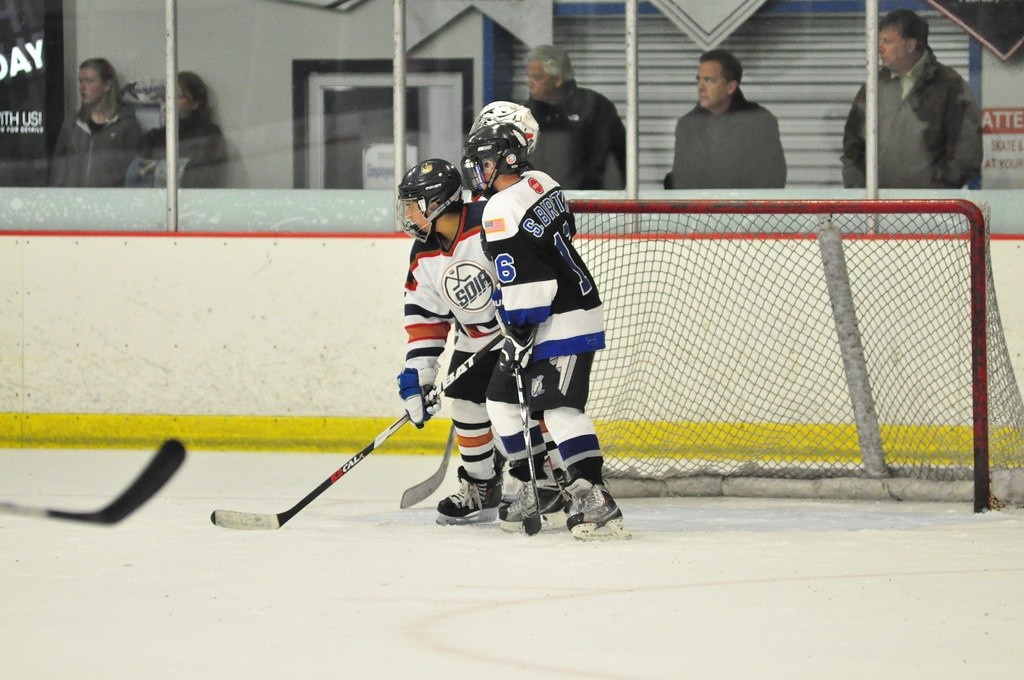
0, 438, 187, 526
399, 421, 458, 511
209, 332, 506, 532
513, 365, 543, 536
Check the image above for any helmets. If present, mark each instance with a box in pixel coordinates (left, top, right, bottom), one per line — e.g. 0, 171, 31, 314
463, 99, 540, 174
397, 158, 465, 213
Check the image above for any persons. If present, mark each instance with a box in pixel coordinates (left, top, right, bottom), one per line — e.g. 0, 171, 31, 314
841, 11, 984, 189
522, 45, 626, 189
397, 157, 567, 526
672, 50, 787, 188
142, 71, 226, 189
469, 102, 538, 201
48, 59, 142, 189
463, 123, 630, 542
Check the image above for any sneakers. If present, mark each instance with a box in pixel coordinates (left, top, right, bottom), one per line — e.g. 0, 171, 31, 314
435, 465, 502, 527
499, 454, 572, 522
560, 475, 632, 542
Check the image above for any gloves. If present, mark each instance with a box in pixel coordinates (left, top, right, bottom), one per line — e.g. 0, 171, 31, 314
395, 361, 442, 430
498, 325, 538, 377
